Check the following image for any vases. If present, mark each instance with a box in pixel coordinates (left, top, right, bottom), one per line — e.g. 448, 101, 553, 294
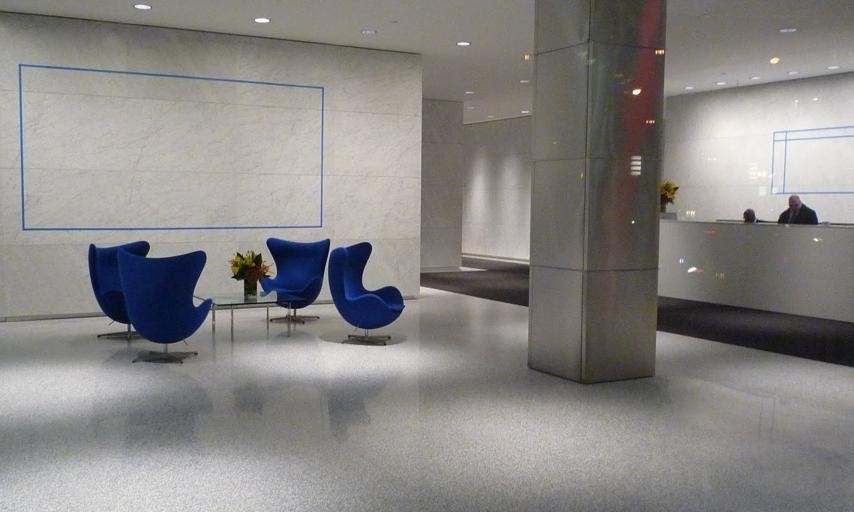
660, 197, 667, 212
244, 279, 258, 303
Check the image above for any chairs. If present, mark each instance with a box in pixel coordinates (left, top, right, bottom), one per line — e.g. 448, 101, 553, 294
88, 240, 150, 341
266, 238, 331, 325
327, 241, 405, 347
117, 245, 212, 365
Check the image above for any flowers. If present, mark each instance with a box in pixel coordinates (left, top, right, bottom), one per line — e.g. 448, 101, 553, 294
660, 179, 679, 205
229, 250, 272, 294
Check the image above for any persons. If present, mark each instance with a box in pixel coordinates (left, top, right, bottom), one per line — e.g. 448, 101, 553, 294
777, 195, 819, 225
743, 209, 766, 223
